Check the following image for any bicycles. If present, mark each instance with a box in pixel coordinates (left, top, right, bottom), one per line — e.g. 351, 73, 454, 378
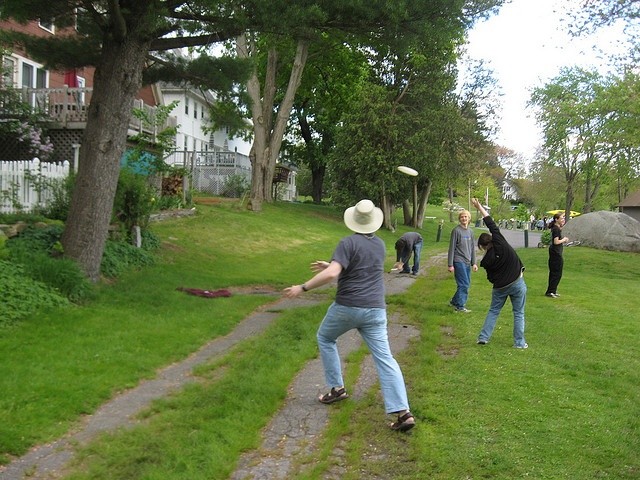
563, 240, 580, 247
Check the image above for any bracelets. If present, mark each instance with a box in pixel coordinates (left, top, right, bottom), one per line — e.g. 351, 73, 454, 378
301, 284, 306, 292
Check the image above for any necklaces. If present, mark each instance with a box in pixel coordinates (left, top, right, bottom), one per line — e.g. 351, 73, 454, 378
365, 235, 374, 239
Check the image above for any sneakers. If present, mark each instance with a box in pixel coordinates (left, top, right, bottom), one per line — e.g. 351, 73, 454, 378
544, 293, 555, 297
514, 343, 528, 348
414, 271, 418, 275
477, 340, 487, 344
555, 294, 560, 296
455, 308, 471, 313
449, 302, 466, 307
399, 270, 409, 273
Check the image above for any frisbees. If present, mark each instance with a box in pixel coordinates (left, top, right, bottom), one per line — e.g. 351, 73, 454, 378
398, 166, 418, 176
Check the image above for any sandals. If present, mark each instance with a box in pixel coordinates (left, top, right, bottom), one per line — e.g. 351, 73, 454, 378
319, 387, 348, 403
390, 412, 415, 432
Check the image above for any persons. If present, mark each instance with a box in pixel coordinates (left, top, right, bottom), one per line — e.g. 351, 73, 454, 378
283, 199, 415, 430
472, 198, 528, 350
394, 232, 423, 276
537, 218, 543, 230
545, 212, 569, 298
544, 216, 547, 229
447, 210, 478, 313
529, 214, 535, 230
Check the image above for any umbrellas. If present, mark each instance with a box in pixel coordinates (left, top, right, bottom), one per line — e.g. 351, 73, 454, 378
546, 211, 580, 216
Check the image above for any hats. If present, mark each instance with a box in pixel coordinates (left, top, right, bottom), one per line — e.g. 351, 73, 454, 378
344, 199, 384, 234
